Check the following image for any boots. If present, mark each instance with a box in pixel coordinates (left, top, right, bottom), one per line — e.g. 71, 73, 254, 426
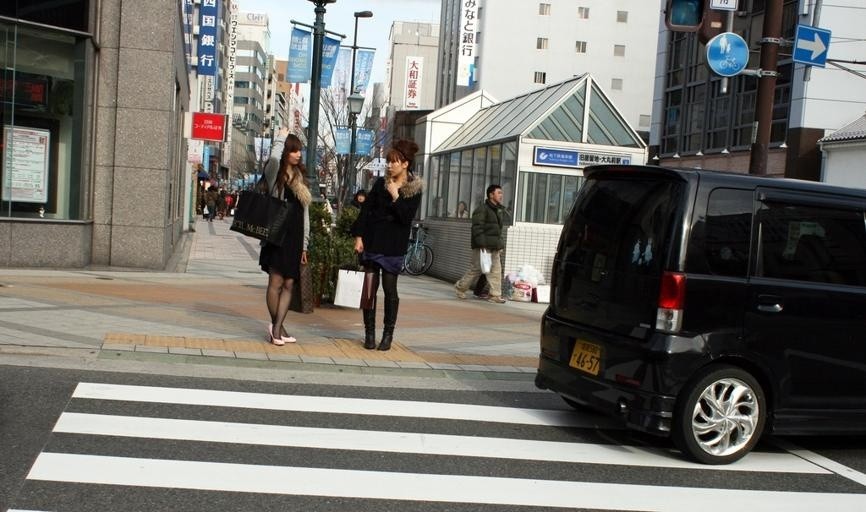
364, 295, 376, 348
376, 297, 399, 351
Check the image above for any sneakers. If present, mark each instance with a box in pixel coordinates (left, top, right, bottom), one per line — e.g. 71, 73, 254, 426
488, 294, 506, 303
453, 282, 467, 298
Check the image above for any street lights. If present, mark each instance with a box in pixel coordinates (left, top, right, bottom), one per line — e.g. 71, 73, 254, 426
252, 159, 259, 186
347, 93, 364, 205
347, 11, 374, 128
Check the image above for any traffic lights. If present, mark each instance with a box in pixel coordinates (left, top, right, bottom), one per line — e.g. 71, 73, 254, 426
666, 1, 708, 32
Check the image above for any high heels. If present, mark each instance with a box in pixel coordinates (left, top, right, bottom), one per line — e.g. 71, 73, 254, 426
266, 324, 296, 346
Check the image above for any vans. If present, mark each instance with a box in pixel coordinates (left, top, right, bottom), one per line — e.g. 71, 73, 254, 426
532, 162, 865, 466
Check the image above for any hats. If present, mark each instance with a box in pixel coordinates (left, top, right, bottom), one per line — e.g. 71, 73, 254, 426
394, 138, 420, 160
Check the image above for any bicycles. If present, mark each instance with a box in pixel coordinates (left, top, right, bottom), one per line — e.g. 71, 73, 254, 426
401, 224, 435, 275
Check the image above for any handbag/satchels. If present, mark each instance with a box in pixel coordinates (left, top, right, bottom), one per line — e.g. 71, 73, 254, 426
288, 261, 313, 314
228, 188, 292, 248
333, 269, 375, 311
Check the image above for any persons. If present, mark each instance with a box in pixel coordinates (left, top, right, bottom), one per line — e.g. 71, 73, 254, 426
431, 197, 448, 216
455, 185, 512, 302
351, 190, 368, 208
352, 140, 424, 350
201, 185, 256, 221
449, 201, 469, 218
320, 193, 333, 233
506, 200, 512, 213
259, 128, 312, 345
